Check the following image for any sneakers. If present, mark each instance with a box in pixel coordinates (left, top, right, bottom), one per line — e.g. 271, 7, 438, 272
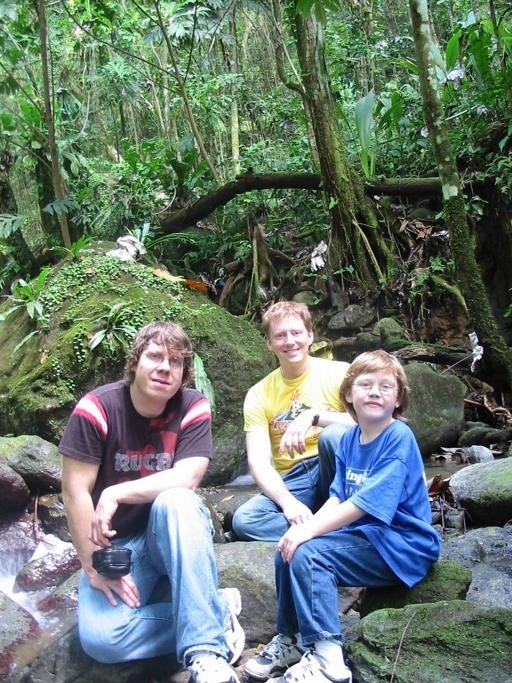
243, 633, 353, 682
217, 586, 246, 665
187, 651, 239, 683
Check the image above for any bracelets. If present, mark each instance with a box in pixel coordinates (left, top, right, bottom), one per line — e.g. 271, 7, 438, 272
308, 408, 320, 428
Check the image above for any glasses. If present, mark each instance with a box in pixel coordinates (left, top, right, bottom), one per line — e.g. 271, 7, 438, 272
349, 380, 400, 395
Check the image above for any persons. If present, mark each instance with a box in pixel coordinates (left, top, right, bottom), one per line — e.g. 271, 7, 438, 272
233, 300, 356, 540
243, 349, 440, 683
54, 320, 246, 682
